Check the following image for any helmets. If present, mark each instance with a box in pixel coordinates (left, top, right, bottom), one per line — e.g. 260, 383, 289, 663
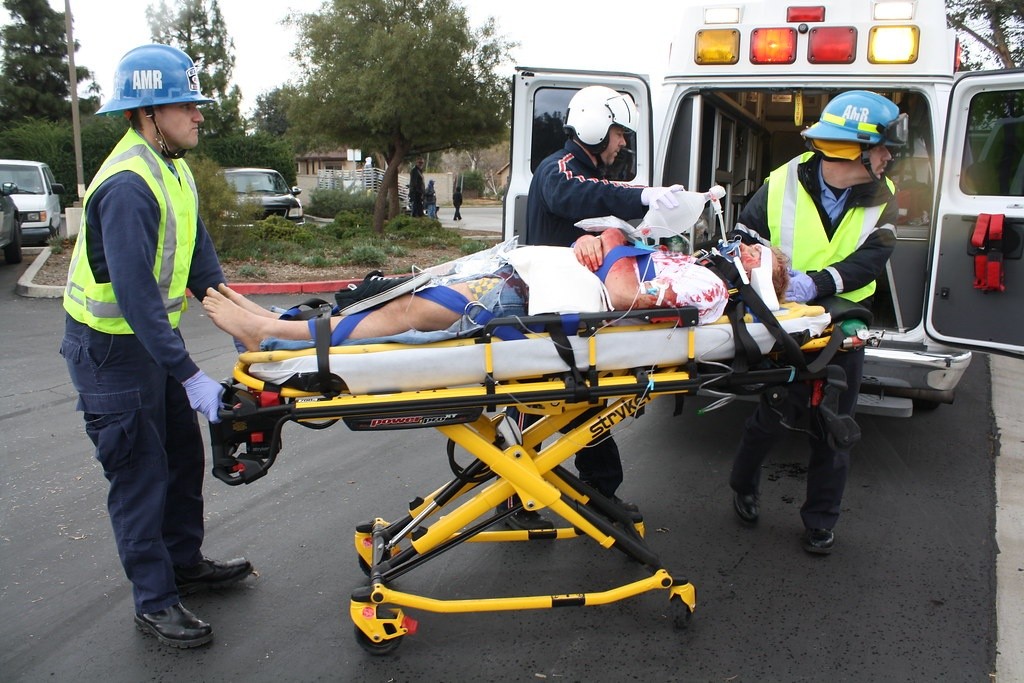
563, 86, 639, 145
801, 89, 900, 144
94, 43, 216, 115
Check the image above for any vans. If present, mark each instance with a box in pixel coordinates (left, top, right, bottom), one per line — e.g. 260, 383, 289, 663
0, 159, 65, 243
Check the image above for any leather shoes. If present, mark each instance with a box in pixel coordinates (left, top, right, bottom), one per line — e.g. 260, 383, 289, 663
803, 529, 835, 553
607, 495, 639, 514
174, 555, 254, 597
495, 505, 555, 529
733, 491, 760, 528
135, 602, 214, 649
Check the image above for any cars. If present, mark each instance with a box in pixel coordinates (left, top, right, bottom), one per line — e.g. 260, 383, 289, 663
218, 168, 304, 229
0, 183, 22, 263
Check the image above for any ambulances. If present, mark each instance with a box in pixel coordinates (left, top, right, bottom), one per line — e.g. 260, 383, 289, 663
501, 0, 1024, 418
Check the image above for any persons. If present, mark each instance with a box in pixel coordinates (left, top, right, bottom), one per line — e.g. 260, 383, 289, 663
408, 158, 426, 217
58, 46, 253, 648
424, 179, 438, 219
202, 229, 788, 353
726, 91, 909, 555
493, 85, 684, 532
453, 186, 463, 220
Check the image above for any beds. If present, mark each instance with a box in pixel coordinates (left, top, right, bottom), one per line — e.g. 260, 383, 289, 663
210, 293, 873, 656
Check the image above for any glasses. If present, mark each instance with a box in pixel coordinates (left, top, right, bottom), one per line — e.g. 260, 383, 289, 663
416, 160, 424, 163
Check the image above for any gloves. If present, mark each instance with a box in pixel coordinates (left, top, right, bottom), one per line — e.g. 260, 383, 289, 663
641, 184, 684, 210
182, 369, 225, 426
232, 334, 247, 354
783, 269, 818, 303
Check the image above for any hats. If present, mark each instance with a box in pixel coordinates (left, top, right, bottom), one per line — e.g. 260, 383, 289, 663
429, 179, 436, 182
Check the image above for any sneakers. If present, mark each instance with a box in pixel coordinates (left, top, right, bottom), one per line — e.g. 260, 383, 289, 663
335, 270, 431, 316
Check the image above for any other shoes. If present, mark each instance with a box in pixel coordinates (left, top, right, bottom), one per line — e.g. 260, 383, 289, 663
459, 217, 461, 220
454, 219, 457, 220
